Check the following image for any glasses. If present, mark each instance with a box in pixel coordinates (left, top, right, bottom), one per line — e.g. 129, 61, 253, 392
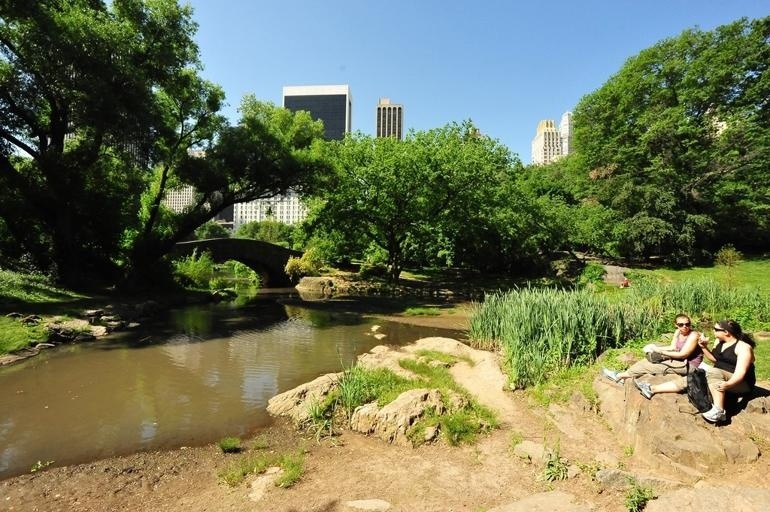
676, 323, 691, 327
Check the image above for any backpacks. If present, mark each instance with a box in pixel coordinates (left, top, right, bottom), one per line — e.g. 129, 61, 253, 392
687, 364, 713, 412
645, 347, 680, 363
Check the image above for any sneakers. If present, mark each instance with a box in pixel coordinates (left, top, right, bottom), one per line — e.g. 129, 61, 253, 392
602, 368, 620, 383
701, 406, 728, 423
633, 378, 654, 400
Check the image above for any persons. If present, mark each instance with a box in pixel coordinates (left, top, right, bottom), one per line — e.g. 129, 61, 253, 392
602, 313, 704, 386
621, 277, 631, 287
633, 319, 758, 423
